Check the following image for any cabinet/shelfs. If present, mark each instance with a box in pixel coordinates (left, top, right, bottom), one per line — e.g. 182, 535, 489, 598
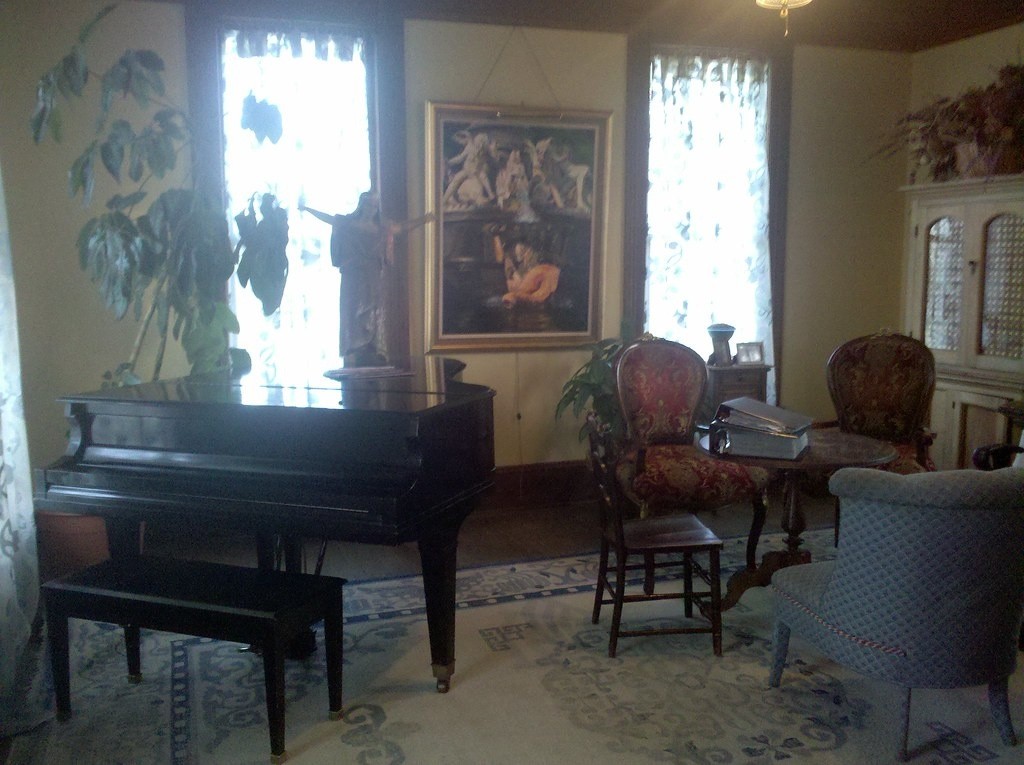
898, 177, 1024, 471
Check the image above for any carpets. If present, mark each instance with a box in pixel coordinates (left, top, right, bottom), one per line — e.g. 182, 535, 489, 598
7, 524, 1024, 765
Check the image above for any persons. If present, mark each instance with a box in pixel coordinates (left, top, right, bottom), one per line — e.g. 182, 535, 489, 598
300, 191, 436, 366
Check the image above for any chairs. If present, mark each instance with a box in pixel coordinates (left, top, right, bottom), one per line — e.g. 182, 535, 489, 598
778, 334, 939, 548
599, 336, 770, 572
586, 413, 722, 655
766, 466, 1024, 763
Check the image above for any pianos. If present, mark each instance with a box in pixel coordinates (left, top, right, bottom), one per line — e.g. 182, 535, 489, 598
30, 356, 499, 694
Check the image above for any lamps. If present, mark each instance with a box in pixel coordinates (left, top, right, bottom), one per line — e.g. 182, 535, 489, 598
756, 0, 810, 37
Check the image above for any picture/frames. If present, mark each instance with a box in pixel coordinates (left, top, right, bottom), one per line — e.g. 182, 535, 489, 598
419, 100, 615, 354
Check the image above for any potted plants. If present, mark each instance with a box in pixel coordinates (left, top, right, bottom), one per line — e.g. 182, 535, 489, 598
864, 68, 1024, 186
553, 335, 648, 525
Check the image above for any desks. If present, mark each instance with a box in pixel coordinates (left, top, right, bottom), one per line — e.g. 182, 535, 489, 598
705, 363, 772, 418
697, 430, 898, 615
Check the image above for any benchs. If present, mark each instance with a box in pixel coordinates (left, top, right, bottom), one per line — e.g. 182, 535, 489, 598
30, 553, 347, 758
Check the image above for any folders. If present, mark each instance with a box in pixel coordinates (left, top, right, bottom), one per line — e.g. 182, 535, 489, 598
708, 418, 811, 461
715, 395, 813, 440
